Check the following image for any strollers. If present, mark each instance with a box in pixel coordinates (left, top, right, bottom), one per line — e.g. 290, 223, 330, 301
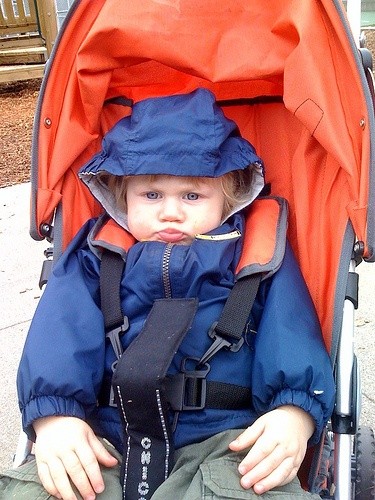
0, 0, 375, 500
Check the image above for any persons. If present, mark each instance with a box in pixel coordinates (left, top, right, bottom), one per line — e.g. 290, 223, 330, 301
1, 87, 338, 500
